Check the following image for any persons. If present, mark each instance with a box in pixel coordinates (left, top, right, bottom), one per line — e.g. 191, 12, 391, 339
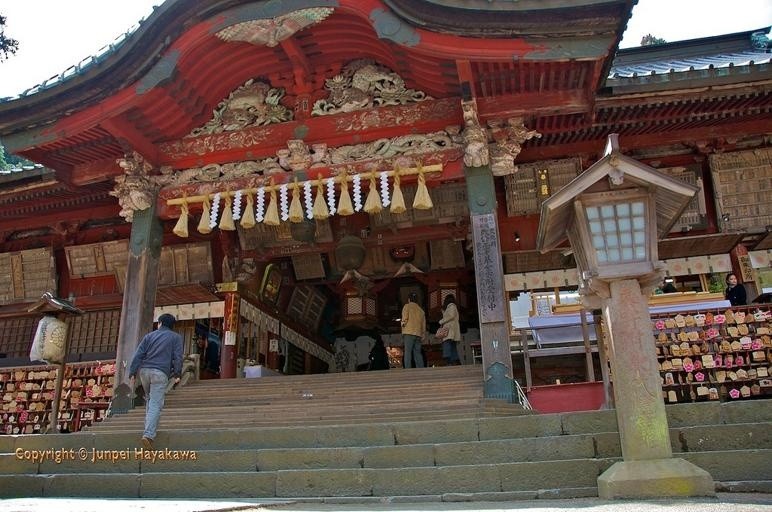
368, 338, 390, 369
127, 312, 184, 451
401, 292, 427, 368
723, 273, 748, 306
437, 293, 462, 366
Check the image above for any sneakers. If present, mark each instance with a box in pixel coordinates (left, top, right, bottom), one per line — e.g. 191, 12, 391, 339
139, 436, 156, 451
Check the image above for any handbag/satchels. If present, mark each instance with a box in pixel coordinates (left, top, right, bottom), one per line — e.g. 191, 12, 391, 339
435, 326, 449, 339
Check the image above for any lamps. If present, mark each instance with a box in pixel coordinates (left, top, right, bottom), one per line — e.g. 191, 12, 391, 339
514, 233, 520, 241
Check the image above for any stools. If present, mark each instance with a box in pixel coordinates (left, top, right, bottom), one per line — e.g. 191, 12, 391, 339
470, 340, 483, 364
